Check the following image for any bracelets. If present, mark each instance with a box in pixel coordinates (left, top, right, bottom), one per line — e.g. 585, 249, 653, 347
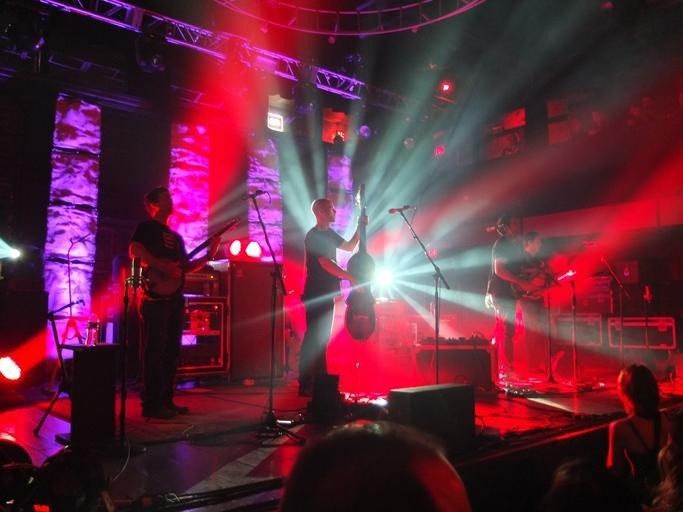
206, 253, 215, 262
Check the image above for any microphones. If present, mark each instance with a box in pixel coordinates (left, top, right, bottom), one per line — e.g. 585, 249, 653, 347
240, 190, 264, 201
131, 256, 142, 289
389, 206, 411, 215
559, 269, 576, 281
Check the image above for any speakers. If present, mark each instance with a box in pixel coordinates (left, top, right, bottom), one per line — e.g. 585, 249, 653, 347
389, 382, 474, 434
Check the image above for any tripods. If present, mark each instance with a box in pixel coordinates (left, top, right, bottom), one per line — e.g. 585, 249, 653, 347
231, 267, 306, 448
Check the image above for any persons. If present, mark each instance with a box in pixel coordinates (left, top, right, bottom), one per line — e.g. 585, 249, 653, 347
297, 198, 368, 395
485, 213, 537, 384
127, 187, 223, 419
282, 421, 472, 511
546, 461, 641, 512
643, 443, 683, 512
605, 362, 672, 496
518, 230, 547, 373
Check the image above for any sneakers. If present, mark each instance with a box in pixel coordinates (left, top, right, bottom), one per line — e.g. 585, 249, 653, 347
298, 384, 313, 395
167, 397, 188, 414
141, 401, 178, 419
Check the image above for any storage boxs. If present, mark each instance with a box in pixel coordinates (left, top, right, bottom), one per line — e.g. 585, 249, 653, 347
554, 313, 604, 347
608, 318, 677, 349
615, 257, 670, 286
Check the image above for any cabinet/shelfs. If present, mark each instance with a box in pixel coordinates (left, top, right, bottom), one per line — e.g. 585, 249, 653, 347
55, 342, 120, 450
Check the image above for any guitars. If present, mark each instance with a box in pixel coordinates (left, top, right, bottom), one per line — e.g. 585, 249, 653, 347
344, 183, 376, 339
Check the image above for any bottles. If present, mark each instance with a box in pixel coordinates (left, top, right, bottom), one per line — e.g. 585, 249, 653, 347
86, 321, 100, 346
